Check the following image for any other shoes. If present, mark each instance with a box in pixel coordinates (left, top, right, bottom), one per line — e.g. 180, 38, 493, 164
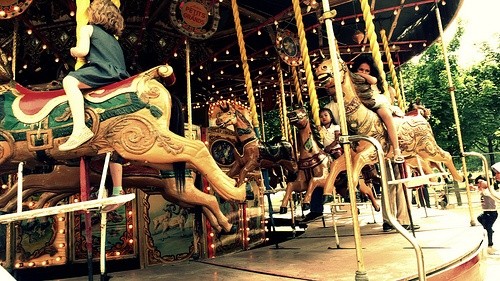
299, 211, 323, 224
487, 247, 494, 255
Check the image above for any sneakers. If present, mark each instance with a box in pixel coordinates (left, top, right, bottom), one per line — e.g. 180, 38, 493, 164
59, 126, 93, 151
101, 190, 126, 213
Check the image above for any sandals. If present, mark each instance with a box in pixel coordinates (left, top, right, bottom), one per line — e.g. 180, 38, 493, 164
394, 153, 404, 163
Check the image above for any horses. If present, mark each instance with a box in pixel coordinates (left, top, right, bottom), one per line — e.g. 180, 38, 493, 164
279, 104, 381, 214
0, 158, 237, 235
316, 38, 473, 205
0, 48, 256, 201
215, 102, 298, 187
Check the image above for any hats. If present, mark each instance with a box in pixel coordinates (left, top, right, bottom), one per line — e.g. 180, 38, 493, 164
491, 161, 500, 173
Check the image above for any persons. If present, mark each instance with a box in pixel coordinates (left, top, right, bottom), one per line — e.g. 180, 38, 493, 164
491, 162, 500, 181
418, 184, 430, 207
382, 87, 419, 232
59, 0, 130, 213
468, 174, 498, 254
438, 190, 447, 209
318, 109, 342, 158
347, 53, 405, 164
314, 87, 340, 132
